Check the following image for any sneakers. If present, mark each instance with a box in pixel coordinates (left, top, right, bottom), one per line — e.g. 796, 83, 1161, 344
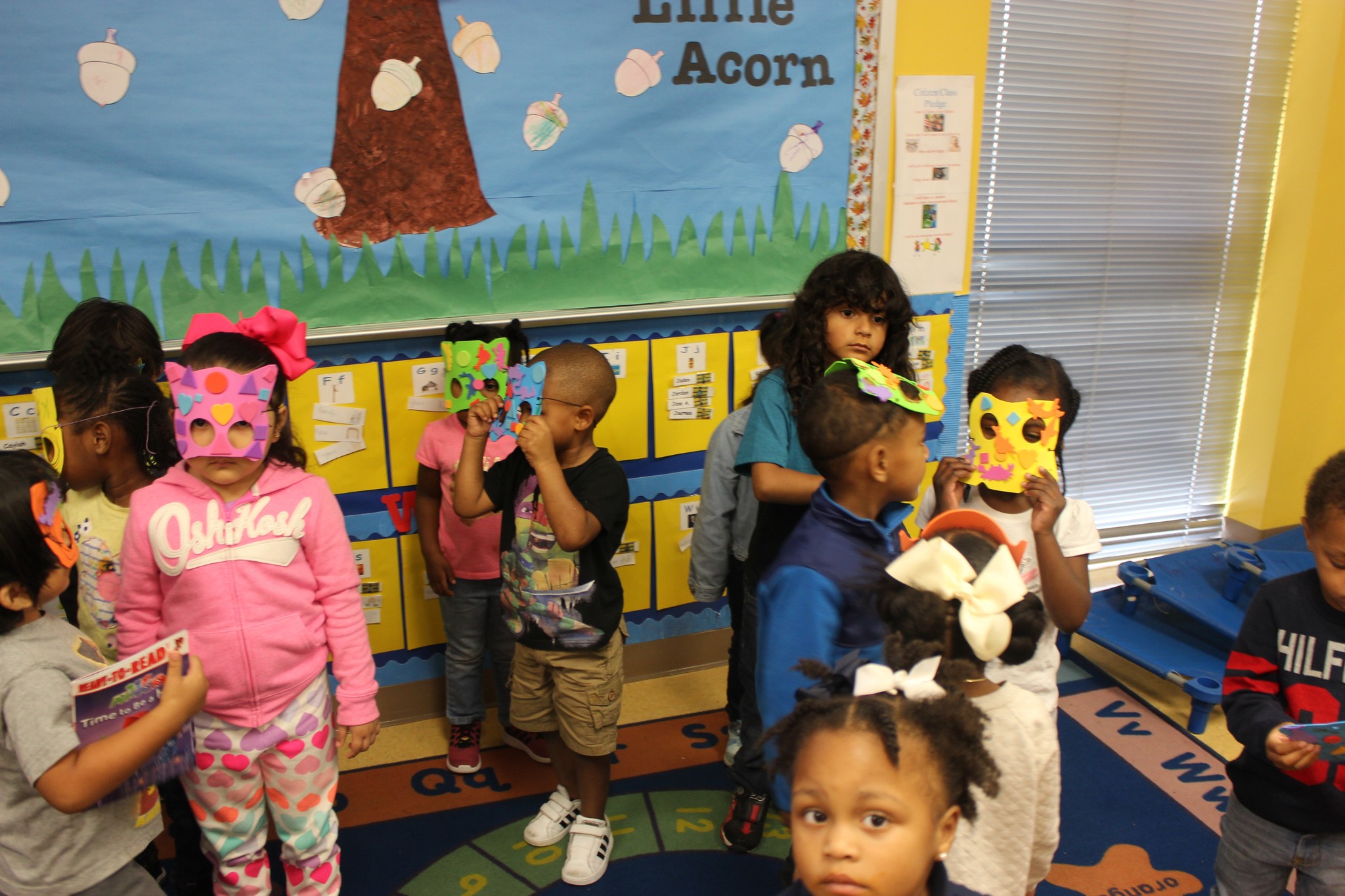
445, 718, 481, 773
720, 785, 769, 852
523, 784, 582, 846
725, 719, 744, 767
502, 723, 551, 764
561, 814, 613, 886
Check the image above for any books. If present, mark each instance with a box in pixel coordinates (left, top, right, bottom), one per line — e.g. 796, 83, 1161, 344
68, 629, 198, 801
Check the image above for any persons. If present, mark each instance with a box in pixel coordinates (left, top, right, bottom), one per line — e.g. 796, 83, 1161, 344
1213, 450, 1345, 896
686, 249, 1103, 896
0, 295, 379, 896
416, 317, 628, 887
